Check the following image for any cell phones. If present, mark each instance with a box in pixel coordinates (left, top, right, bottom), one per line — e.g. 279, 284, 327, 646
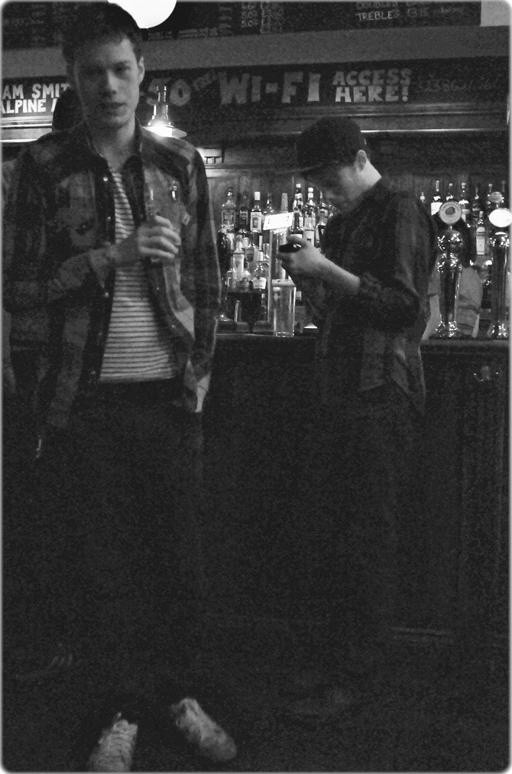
279, 243, 302, 252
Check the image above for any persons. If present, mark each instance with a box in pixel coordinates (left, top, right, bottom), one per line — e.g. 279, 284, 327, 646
3, 2, 238, 773
275, 118, 439, 726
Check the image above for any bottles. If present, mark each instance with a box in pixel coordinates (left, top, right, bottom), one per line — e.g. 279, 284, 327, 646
430, 178, 508, 256
219, 182, 329, 293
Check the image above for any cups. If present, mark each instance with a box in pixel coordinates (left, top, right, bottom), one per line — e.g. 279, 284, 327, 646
270, 280, 298, 340
144, 184, 182, 264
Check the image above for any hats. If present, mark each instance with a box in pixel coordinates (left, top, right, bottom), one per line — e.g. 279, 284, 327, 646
275, 118, 367, 175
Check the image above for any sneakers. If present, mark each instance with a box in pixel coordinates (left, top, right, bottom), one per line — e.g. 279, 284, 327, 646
168, 697, 236, 763
278, 695, 365, 726
88, 712, 138, 772
